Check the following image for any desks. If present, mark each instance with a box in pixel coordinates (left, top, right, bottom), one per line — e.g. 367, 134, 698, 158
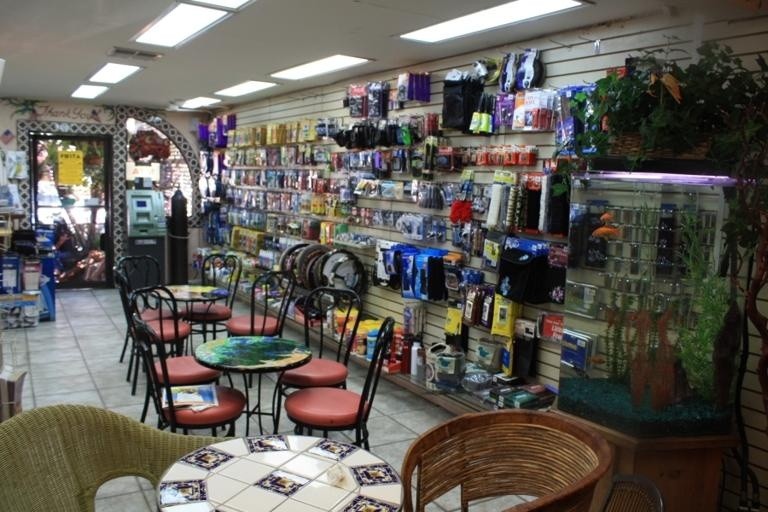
193, 336, 312, 436
156, 434, 405, 512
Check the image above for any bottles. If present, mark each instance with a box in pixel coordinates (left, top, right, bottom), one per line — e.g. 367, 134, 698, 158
409, 339, 426, 378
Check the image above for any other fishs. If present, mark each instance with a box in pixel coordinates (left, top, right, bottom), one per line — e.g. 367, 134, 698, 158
592, 224, 625, 241
591, 357, 606, 365
569, 364, 589, 379
600, 212, 614, 225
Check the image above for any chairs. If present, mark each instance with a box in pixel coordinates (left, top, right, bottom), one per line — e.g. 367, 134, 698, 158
284, 317, 395, 442
598, 471, 665, 512
401, 408, 617, 512
114, 254, 296, 388
0, 405, 240, 512
129, 315, 246, 439
274, 285, 362, 432
61, 226, 89, 270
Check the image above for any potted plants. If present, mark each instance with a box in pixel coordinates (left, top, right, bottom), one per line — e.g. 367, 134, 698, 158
553, 42, 768, 260
